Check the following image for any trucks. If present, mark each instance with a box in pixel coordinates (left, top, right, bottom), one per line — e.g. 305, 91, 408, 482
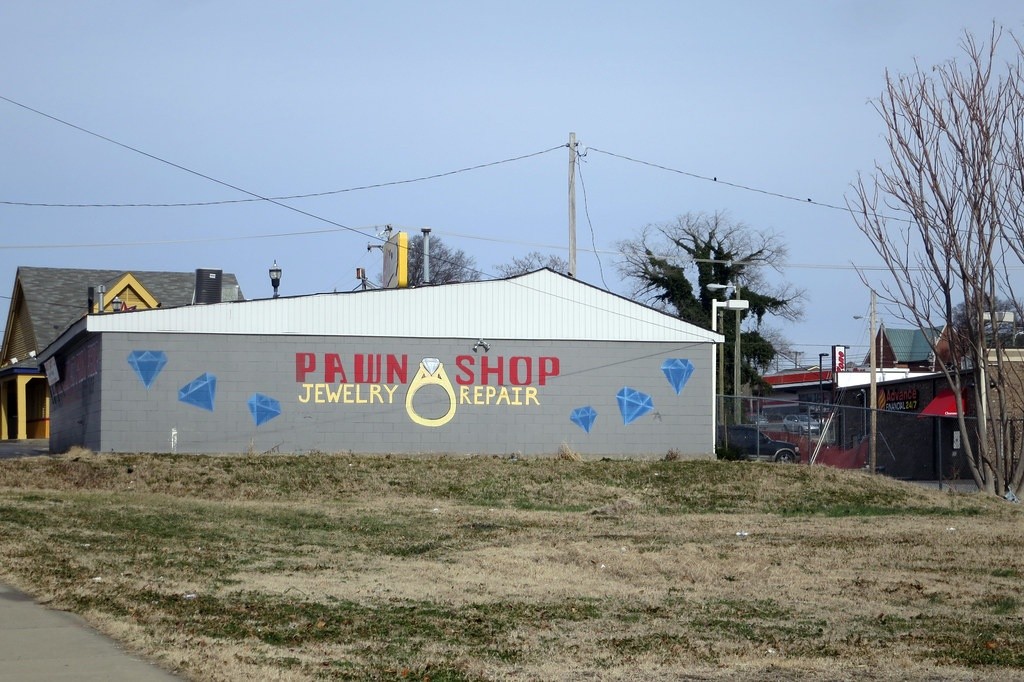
760, 402, 810, 423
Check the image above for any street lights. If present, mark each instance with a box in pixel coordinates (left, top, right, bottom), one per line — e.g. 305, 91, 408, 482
706, 282, 741, 426
854, 314, 884, 372
711, 300, 749, 454
818, 352, 829, 436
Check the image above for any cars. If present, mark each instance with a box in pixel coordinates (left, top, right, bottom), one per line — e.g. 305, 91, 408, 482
806, 405, 835, 433
751, 414, 769, 431
782, 413, 819, 436
714, 423, 802, 465
